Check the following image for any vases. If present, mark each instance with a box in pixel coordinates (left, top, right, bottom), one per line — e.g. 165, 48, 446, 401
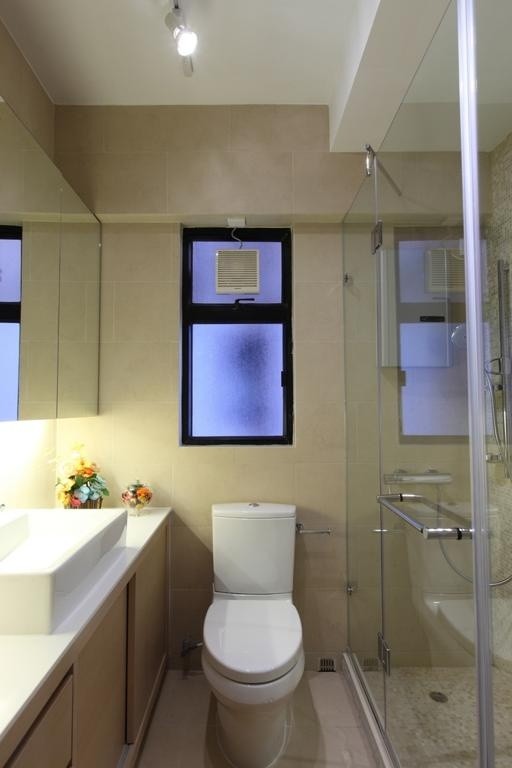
63, 498, 103, 510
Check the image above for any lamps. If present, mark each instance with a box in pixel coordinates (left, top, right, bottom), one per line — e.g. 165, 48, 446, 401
164, 2, 204, 64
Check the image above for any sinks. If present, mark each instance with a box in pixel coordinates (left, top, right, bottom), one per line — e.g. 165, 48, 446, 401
0, 508, 128, 634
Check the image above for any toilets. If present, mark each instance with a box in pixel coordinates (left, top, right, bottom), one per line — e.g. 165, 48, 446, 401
401, 504, 512, 760
200, 502, 306, 767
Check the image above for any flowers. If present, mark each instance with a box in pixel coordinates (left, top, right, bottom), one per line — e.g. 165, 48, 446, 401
53, 440, 114, 506
120, 478, 153, 511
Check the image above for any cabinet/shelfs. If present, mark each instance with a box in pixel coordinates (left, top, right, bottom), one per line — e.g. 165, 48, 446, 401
1, 669, 80, 768
75, 526, 171, 768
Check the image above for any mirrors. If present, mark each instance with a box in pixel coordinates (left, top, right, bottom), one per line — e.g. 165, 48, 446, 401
1, 98, 105, 426
